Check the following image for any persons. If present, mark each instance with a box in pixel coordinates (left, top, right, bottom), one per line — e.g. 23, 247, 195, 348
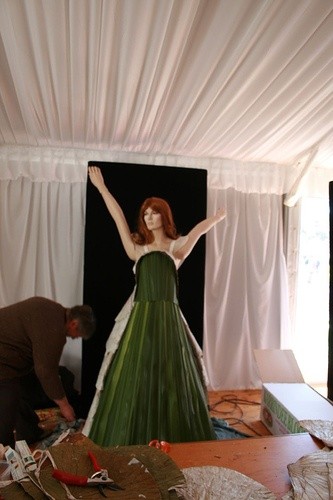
0, 295, 96, 448
82, 167, 227, 449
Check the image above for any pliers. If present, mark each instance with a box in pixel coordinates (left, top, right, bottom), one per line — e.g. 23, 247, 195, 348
52, 451, 126, 498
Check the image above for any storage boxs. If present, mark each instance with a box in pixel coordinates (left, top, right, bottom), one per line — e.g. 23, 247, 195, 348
260, 382, 333, 436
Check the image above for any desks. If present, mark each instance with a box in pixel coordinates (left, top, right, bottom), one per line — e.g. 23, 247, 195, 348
0, 430, 320, 500
